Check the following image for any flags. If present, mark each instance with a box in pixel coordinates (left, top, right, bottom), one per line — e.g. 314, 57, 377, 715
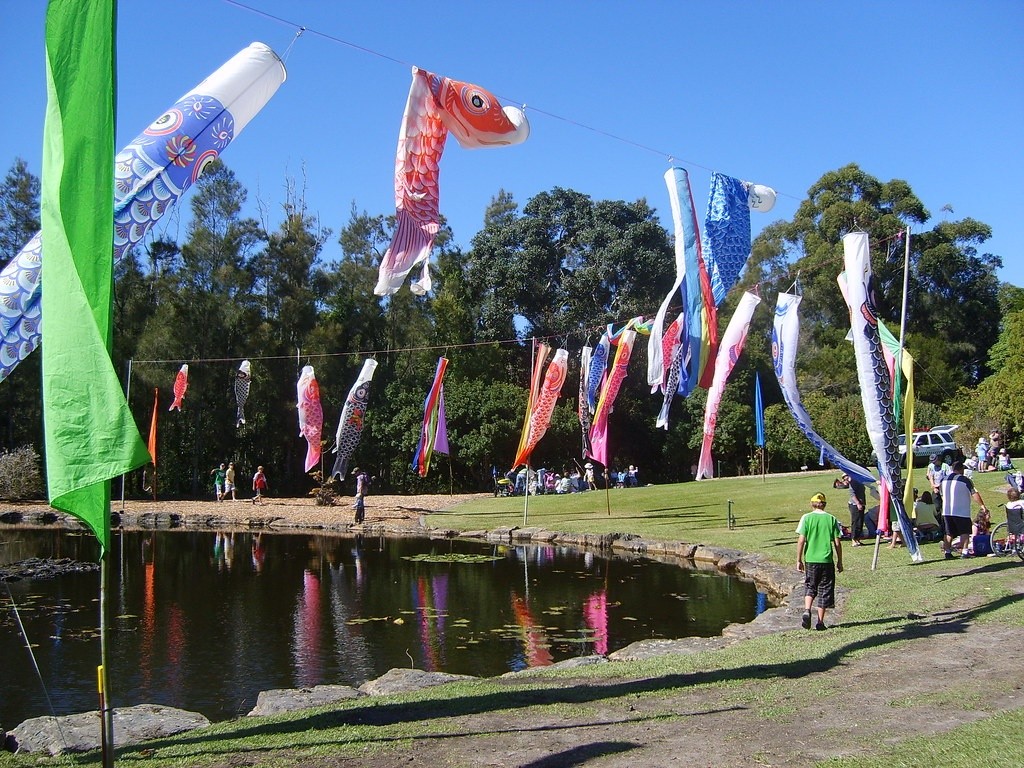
44, 0, 151, 552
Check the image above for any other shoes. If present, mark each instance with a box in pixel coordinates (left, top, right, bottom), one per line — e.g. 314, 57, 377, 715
961, 549, 976, 558
816, 622, 828, 630
801, 609, 811, 629
886, 545, 895, 549
979, 470, 985, 472
944, 553, 955, 559
899, 544, 906, 548
851, 539, 863, 546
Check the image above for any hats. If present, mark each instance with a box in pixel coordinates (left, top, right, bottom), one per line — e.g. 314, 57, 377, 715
811, 492, 827, 503
351, 467, 359, 474
999, 448, 1006, 453
929, 453, 939, 463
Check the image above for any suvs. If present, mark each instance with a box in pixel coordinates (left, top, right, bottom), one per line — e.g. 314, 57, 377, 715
871, 425, 959, 470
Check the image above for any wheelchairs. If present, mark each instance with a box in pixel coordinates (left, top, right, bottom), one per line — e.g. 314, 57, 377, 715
989, 503, 1024, 561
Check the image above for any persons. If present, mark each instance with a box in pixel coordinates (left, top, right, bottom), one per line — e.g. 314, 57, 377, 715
331, 458, 639, 523
209, 529, 369, 602
833, 464, 866, 547
963, 430, 1014, 473
143, 462, 268, 504
797, 492, 843, 630
919, 436, 939, 445
963, 360, 978, 389
940, 461, 991, 559
864, 479, 940, 549
927, 454, 951, 536
1005, 471, 1024, 545
